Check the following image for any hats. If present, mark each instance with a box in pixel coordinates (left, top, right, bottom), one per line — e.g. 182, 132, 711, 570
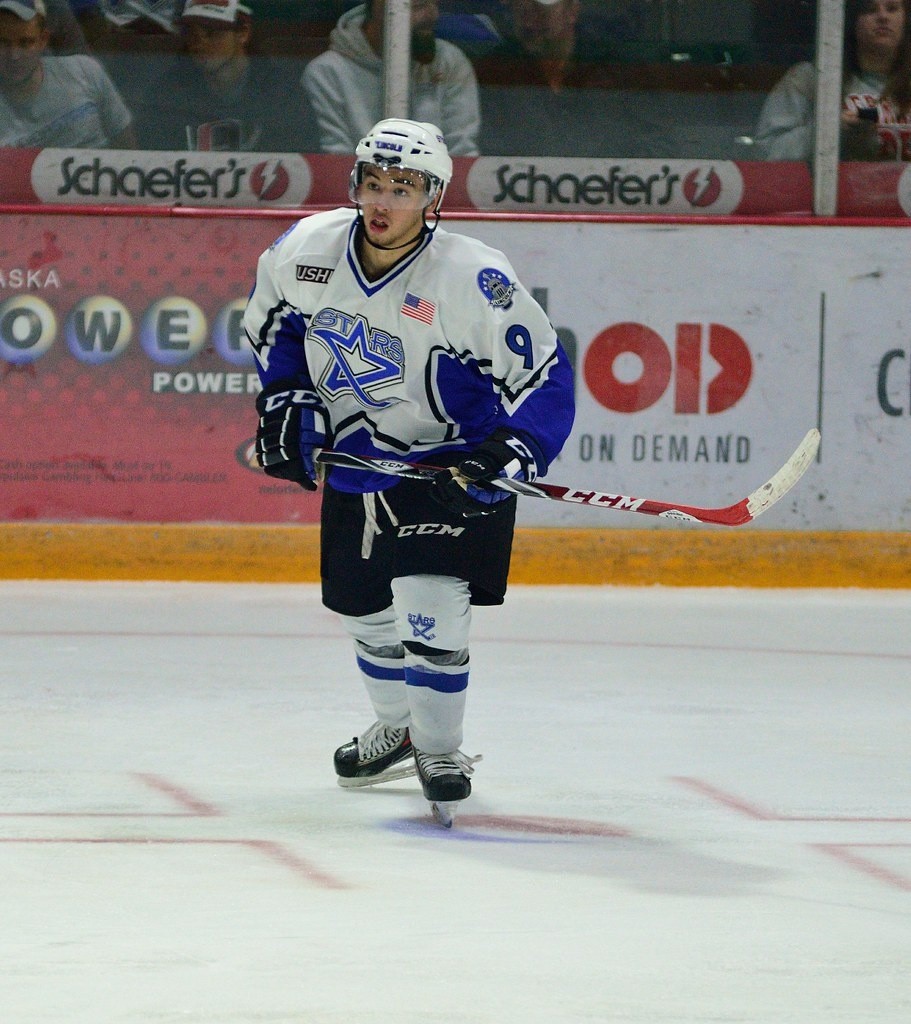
175, 0, 253, 29
0, 0, 46, 21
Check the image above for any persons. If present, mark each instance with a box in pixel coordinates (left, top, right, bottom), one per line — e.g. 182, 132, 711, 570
236, 117, 577, 833
0, 0, 910, 164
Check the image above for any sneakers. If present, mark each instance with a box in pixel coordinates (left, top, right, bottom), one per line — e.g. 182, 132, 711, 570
412, 745, 483, 829
334, 720, 417, 787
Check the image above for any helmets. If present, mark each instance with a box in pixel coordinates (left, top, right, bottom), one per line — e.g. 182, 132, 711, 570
354, 118, 453, 215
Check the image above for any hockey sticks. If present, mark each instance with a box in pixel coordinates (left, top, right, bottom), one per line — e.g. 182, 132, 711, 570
309, 426, 822, 529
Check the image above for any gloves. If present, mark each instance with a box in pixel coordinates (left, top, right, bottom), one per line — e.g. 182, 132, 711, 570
426, 426, 548, 518
254, 374, 334, 492
